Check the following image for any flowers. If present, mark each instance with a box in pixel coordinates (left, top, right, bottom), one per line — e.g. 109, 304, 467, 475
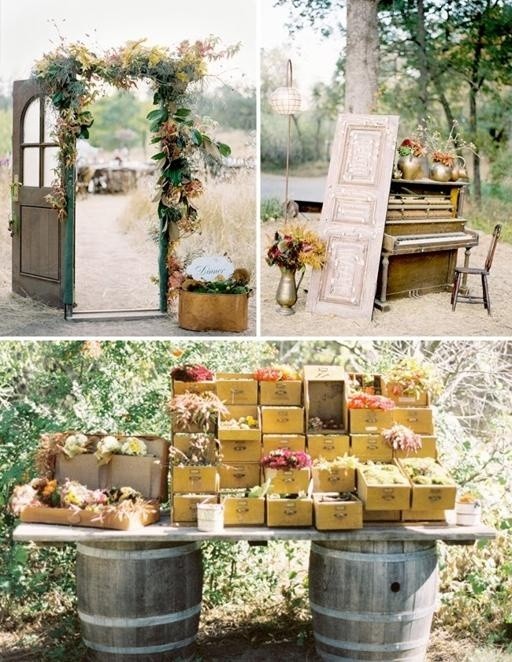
427, 120, 482, 165
31, 18, 240, 326
264, 220, 329, 273
396, 115, 432, 158
7, 434, 165, 523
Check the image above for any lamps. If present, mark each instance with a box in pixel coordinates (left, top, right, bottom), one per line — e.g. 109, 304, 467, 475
269, 60, 301, 225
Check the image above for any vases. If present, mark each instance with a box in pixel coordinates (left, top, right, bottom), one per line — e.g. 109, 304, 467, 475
179, 289, 248, 332
276, 264, 306, 316
168, 267, 250, 293
432, 163, 468, 182
398, 156, 420, 180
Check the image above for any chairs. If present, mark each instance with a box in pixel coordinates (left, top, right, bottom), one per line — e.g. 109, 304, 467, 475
451, 224, 501, 315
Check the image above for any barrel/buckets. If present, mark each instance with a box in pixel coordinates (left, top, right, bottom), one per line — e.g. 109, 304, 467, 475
306, 539, 441, 660
72, 542, 207, 662
